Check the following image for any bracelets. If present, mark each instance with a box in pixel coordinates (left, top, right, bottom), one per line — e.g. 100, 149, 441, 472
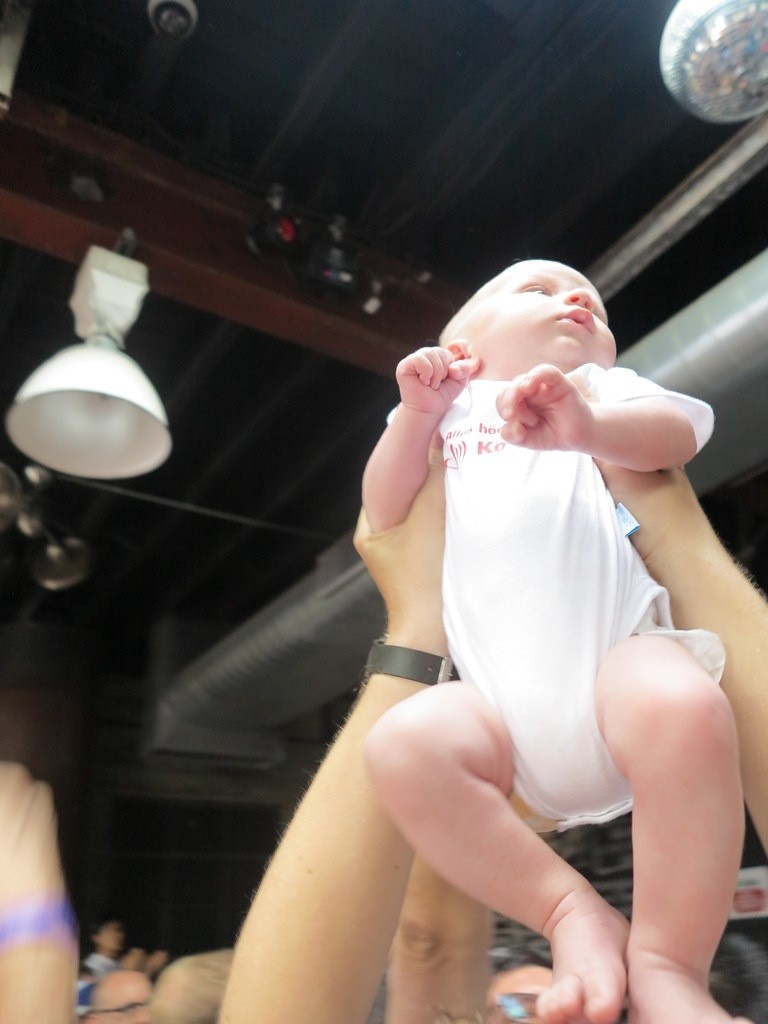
0, 901, 79, 945
363, 639, 459, 686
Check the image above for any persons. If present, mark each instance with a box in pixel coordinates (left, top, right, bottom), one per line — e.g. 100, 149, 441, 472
359, 257, 753, 1024
0, 758, 234, 1024
218, 428, 768, 1024
